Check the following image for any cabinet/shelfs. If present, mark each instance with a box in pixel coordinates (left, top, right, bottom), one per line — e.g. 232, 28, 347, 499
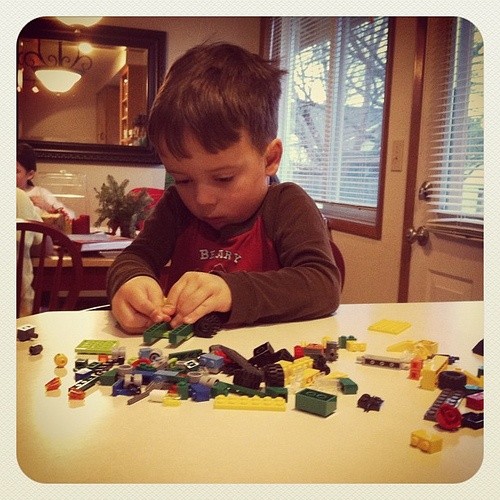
96, 65, 146, 145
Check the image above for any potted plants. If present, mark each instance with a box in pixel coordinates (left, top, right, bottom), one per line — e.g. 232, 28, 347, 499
93, 175, 154, 238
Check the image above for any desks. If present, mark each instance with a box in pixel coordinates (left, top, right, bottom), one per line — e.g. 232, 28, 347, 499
16, 301, 484, 484
32, 255, 115, 292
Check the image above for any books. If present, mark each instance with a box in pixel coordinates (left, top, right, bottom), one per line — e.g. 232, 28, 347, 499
66, 232, 136, 251
99, 250, 124, 259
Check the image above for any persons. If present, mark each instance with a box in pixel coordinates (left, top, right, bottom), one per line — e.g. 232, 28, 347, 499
105, 42, 341, 334
16, 142, 75, 258
128, 114, 147, 147
16, 187, 44, 319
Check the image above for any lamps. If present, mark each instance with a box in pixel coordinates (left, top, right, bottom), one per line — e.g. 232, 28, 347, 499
17, 38, 93, 96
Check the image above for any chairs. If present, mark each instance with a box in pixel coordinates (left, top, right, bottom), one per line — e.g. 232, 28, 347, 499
16, 218, 83, 319
128, 187, 165, 231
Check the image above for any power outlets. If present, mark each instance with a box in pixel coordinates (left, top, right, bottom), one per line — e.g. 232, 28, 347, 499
391, 140, 404, 171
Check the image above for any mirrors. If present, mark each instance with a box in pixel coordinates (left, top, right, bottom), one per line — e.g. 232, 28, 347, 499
17, 16, 163, 165
